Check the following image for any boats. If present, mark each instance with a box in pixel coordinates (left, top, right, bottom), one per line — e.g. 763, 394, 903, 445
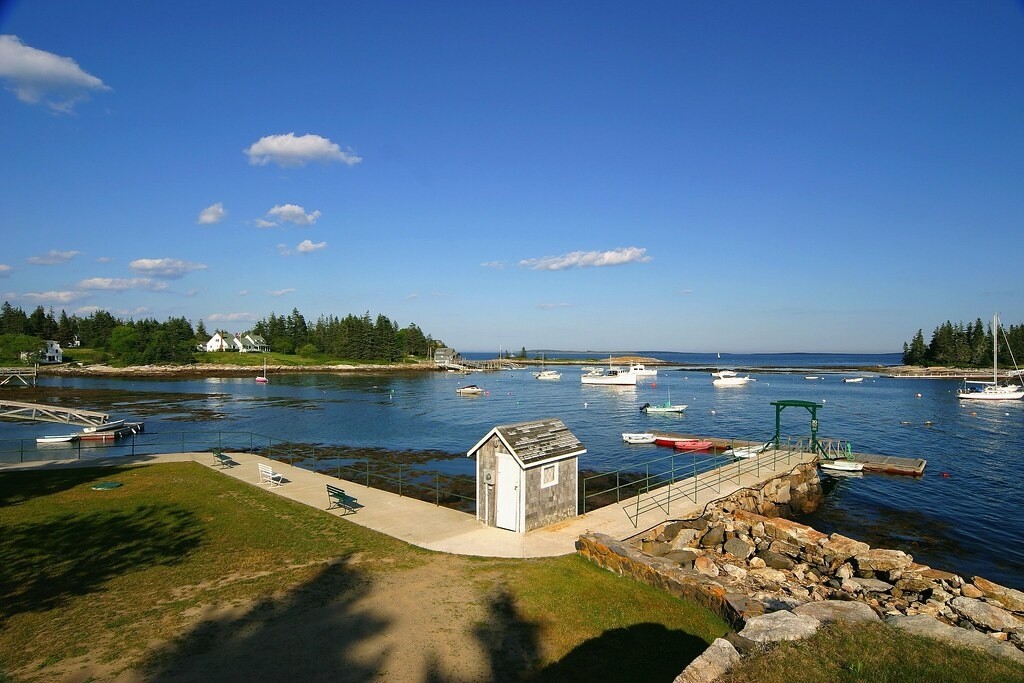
821, 460, 865, 471
622, 433, 713, 450
255, 376, 268, 382
804, 376, 818, 379
580, 354, 657, 385
721, 445, 770, 458
640, 386, 689, 413
954, 312, 1024, 400
83, 419, 125, 433
456, 384, 484, 394
36, 435, 78, 442
841, 377, 864, 383
710, 369, 739, 377
532, 358, 561, 380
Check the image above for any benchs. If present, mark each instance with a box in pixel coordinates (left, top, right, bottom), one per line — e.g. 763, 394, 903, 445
326, 484, 358, 516
211, 447, 233, 470
258, 463, 284, 489
